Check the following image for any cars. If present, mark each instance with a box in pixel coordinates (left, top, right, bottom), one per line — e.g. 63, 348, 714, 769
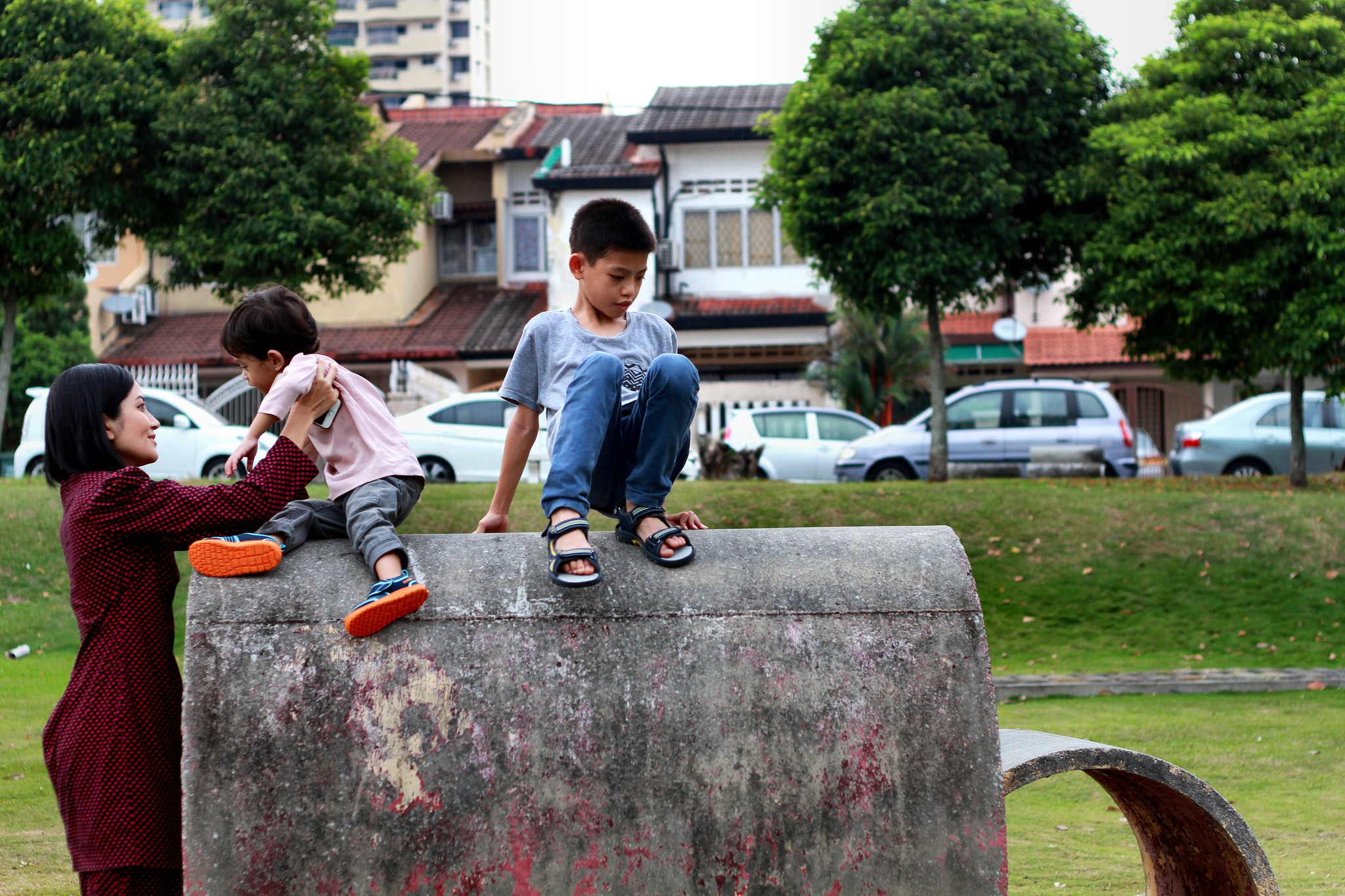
830, 376, 1140, 481
726, 406, 884, 489
11, 386, 279, 484
1166, 390, 1345, 478
1136, 429, 1165, 482
392, 392, 702, 484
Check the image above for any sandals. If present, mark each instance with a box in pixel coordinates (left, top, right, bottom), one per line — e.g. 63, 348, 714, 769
540, 517, 604, 588
614, 499, 695, 567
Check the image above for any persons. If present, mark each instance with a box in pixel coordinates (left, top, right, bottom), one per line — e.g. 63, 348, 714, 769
188, 281, 429, 636
472, 197, 709, 588
43, 358, 338, 896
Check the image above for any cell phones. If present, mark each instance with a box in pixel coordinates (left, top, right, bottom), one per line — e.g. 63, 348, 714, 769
312, 398, 342, 429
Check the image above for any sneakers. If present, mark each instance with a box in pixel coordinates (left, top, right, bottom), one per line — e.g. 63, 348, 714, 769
187, 531, 285, 576
345, 568, 428, 637
